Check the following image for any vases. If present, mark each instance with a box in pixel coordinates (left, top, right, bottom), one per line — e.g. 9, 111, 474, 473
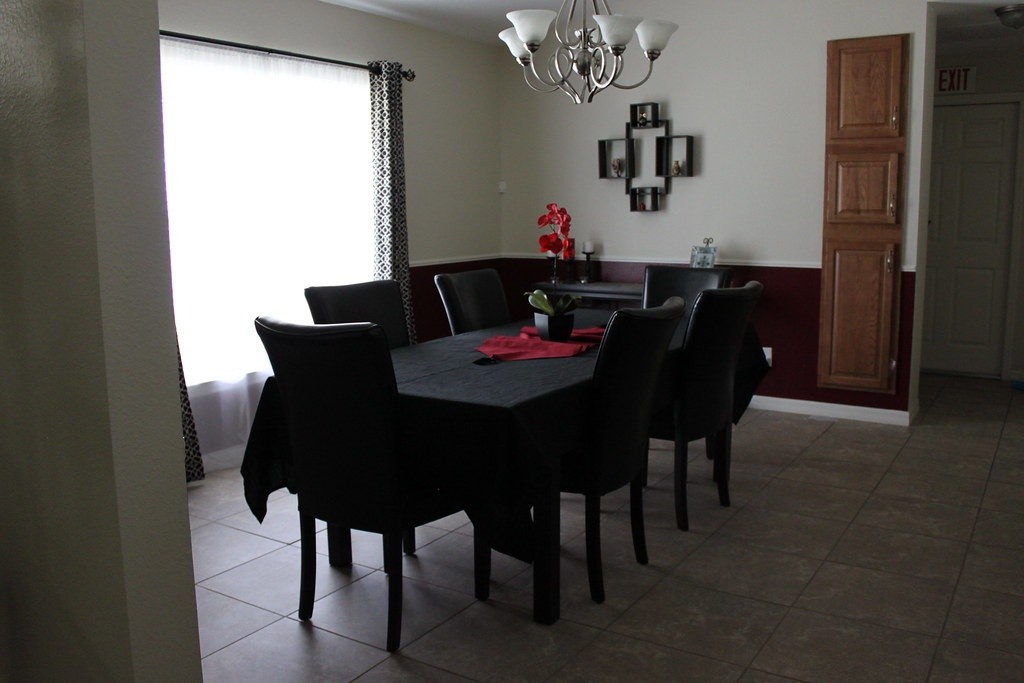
533, 312, 574, 340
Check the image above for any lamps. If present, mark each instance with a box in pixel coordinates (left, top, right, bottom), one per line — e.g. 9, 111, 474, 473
498, 0, 678, 107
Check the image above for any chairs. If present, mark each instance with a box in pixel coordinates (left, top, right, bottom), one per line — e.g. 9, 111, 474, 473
251, 265, 763, 653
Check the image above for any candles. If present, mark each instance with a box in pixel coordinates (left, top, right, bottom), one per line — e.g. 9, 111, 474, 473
583, 240, 594, 252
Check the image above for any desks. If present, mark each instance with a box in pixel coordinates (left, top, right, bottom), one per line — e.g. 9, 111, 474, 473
240, 309, 687, 626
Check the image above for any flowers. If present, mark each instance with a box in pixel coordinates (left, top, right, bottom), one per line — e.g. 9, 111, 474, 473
523, 204, 584, 316
